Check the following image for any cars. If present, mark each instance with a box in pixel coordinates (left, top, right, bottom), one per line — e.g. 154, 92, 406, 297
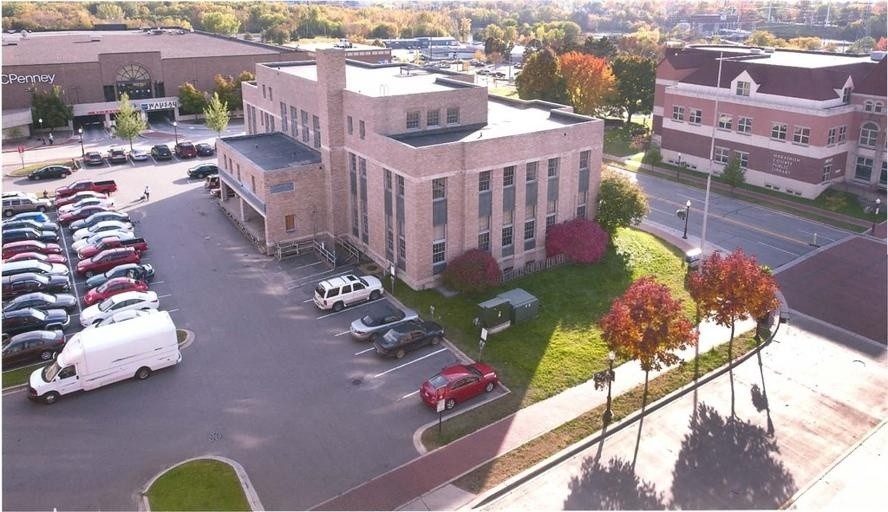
27, 165, 72, 180
420, 364, 497, 410
2, 180, 161, 369
84, 141, 214, 167
187, 163, 236, 199
514, 62, 522, 68
470, 59, 506, 78
312, 273, 445, 359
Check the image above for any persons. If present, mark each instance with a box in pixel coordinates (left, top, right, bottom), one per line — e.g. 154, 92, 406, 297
43, 189, 48, 198
144, 186, 150, 199
48, 131, 54, 144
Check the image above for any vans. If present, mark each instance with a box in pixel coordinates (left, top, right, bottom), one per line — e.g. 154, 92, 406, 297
26, 307, 183, 406
334, 38, 349, 48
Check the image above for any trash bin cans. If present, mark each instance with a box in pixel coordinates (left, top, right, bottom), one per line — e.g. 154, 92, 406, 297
463, 62, 469, 70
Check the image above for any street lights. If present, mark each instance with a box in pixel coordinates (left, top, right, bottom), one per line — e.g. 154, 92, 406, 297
872, 198, 881, 232
682, 200, 691, 239
607, 350, 616, 414
38, 118, 43, 145
677, 152, 682, 181
78, 129, 85, 157
172, 121, 178, 145
